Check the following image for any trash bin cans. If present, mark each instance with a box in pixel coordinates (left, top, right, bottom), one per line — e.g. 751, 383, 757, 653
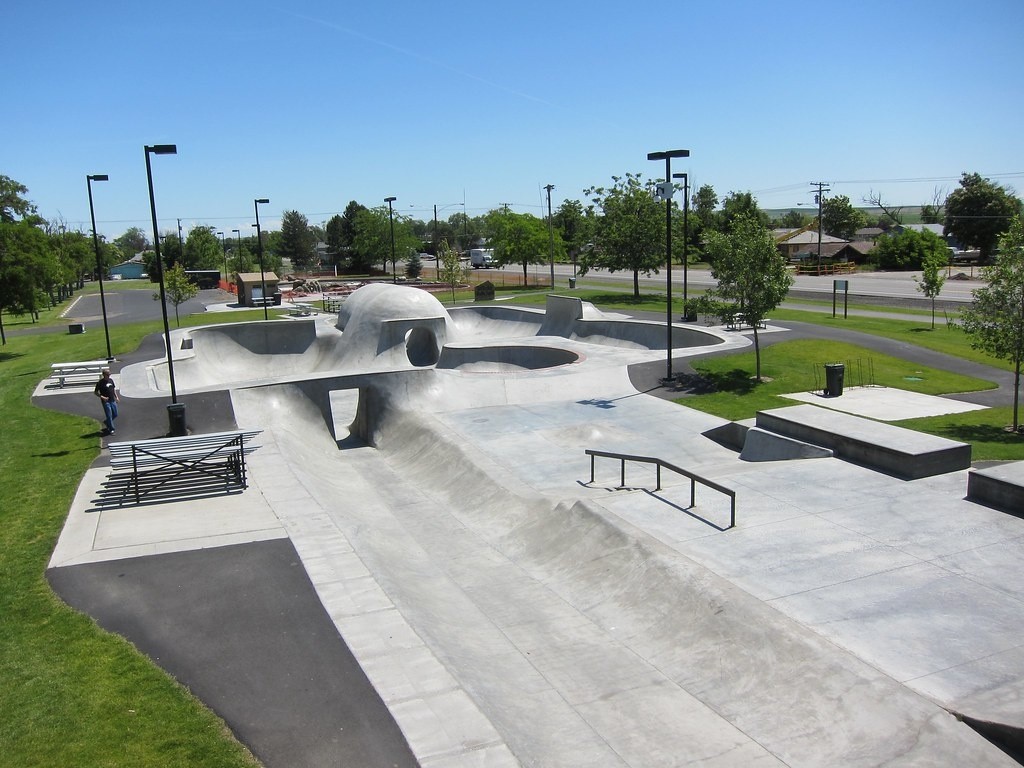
685, 304, 698, 322
569, 278, 576, 288
823, 363, 845, 397
166, 403, 188, 438
273, 292, 282, 305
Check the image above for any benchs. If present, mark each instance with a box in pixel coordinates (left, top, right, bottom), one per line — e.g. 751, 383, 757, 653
726, 318, 771, 331
253, 301, 275, 306
287, 306, 320, 316
54, 366, 110, 381
51, 372, 103, 388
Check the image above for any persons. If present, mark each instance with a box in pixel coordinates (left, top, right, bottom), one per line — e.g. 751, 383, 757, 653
94, 369, 118, 435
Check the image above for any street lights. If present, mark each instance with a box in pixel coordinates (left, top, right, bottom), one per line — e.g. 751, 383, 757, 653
87, 174, 116, 362
646, 149, 691, 388
144, 142, 189, 436
251, 198, 269, 320
797, 202, 822, 265
674, 172, 689, 322
384, 196, 398, 284
217, 232, 228, 283
232, 229, 243, 273
434, 203, 465, 282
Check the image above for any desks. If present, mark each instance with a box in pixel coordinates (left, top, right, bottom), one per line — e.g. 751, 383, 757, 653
252, 297, 274, 305
51, 360, 109, 385
294, 303, 314, 315
726, 311, 761, 328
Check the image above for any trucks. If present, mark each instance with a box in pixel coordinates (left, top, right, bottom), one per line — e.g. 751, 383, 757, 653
471, 248, 501, 270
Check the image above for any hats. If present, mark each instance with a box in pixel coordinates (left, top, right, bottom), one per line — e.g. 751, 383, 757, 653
102, 369, 112, 376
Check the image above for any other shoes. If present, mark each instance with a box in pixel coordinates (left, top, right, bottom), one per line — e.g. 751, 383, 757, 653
109, 429, 114, 434
103, 420, 108, 426
